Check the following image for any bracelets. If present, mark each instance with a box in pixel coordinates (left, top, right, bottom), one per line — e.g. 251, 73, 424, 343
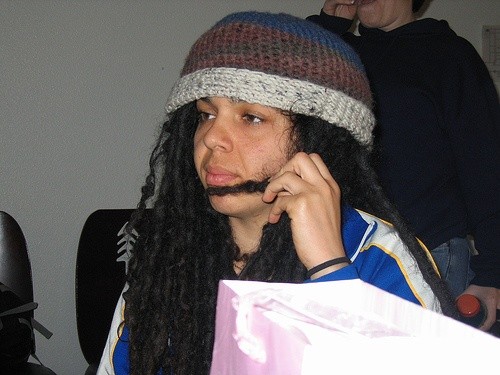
307, 257, 351, 278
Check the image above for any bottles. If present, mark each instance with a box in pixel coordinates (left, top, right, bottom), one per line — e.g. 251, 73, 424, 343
454, 294, 488, 329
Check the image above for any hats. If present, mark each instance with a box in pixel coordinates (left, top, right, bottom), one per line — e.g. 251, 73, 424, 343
164, 11, 377, 156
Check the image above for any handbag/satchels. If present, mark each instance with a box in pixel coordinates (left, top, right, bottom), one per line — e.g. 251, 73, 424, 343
210, 278, 499, 375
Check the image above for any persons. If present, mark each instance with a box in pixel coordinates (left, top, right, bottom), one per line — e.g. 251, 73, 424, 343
305, 1, 500, 331
96, 11, 461, 375
